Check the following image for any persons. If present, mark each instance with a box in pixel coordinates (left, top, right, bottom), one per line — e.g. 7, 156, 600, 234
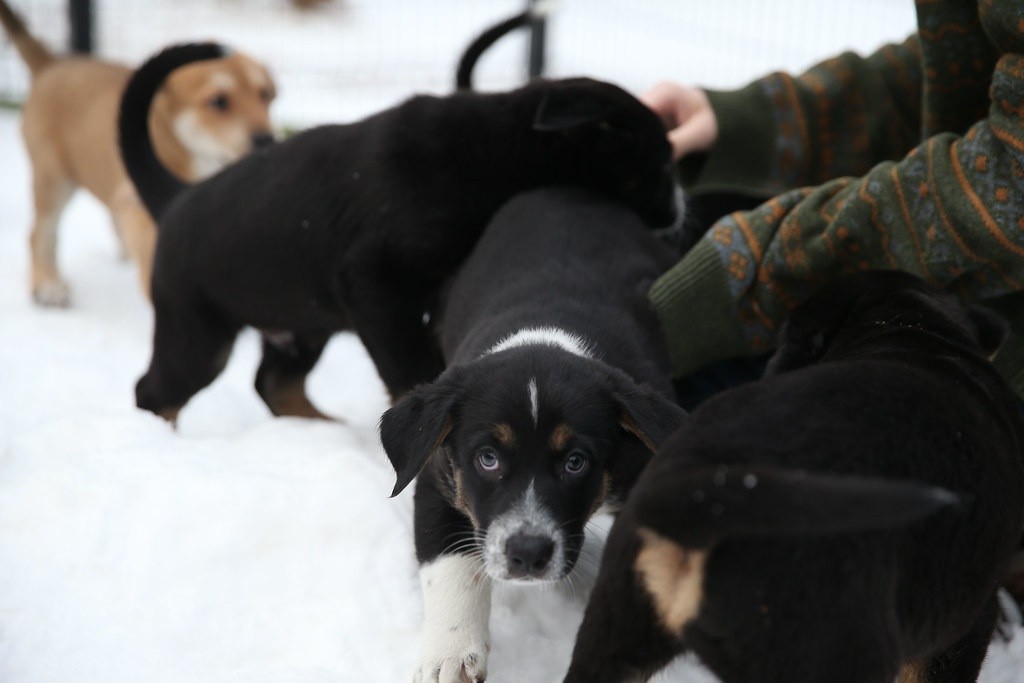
636, 0, 1024, 379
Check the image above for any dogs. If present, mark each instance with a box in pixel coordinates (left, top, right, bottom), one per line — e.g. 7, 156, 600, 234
113, 37, 680, 431
555, 263, 1024, 683
0, 0, 280, 309
377, 179, 686, 683
